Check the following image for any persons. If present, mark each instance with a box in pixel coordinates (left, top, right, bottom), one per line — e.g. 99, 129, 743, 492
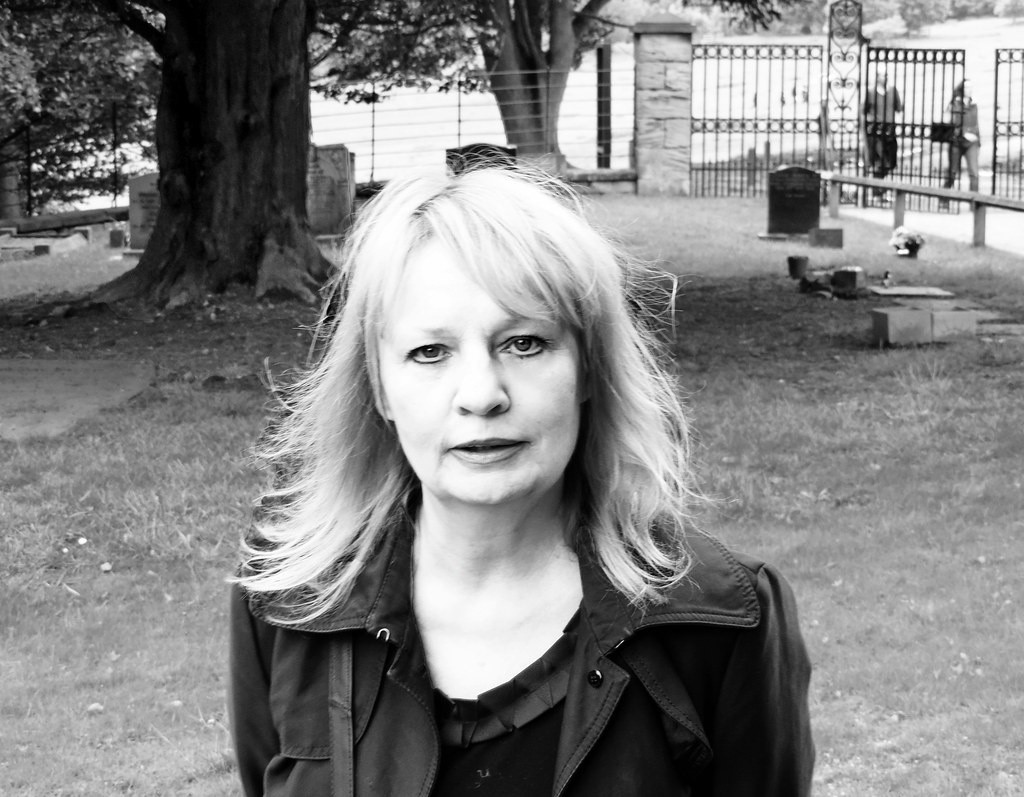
863, 68, 904, 202
939, 78, 981, 210
229, 166, 817, 797
